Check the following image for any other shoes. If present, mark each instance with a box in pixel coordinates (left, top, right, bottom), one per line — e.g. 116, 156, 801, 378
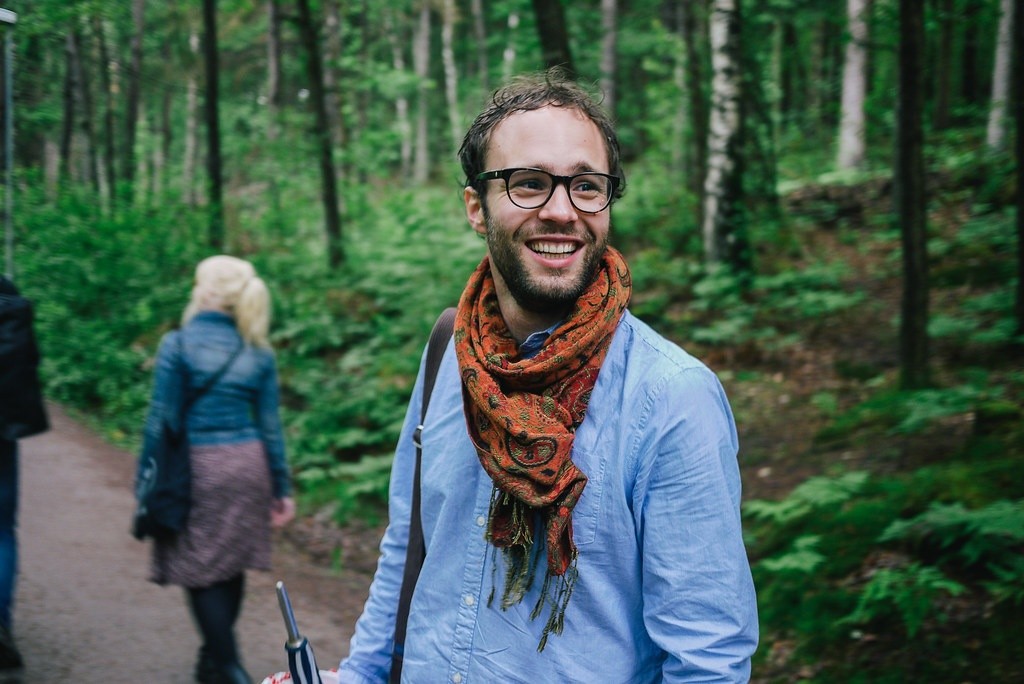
192, 645, 254, 684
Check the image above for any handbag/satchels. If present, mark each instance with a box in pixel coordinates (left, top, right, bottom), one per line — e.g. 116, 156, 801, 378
130, 425, 193, 543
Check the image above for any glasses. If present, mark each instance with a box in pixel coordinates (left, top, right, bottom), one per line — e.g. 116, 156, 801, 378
472, 168, 622, 214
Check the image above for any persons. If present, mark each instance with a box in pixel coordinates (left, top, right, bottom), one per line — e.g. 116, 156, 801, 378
130, 254, 297, 684
336, 65, 760, 684
0, 273, 52, 671
